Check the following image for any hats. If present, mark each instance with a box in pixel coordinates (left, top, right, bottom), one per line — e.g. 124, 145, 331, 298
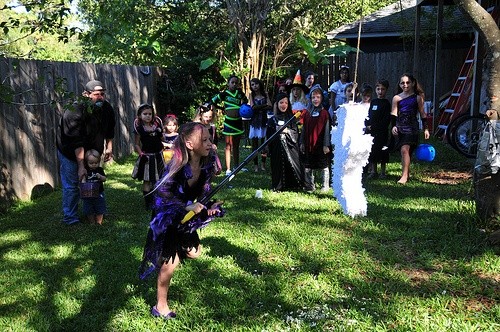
291, 69, 306, 90
85, 81, 108, 92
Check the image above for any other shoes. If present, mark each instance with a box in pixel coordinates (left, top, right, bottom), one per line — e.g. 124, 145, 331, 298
152, 305, 181, 322
226, 170, 234, 177
368, 171, 388, 181
233, 167, 248, 173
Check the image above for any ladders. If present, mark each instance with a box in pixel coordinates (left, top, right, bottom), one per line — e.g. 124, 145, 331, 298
438, 6, 494, 130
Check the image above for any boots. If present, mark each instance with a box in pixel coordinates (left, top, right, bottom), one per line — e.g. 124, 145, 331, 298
143, 189, 157, 210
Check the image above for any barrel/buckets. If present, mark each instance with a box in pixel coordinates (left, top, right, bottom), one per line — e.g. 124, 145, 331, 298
163, 149, 174, 161
78, 180, 99, 200
417, 138, 435, 161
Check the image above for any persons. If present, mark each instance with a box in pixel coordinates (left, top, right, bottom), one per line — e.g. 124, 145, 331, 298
359, 85, 373, 104
194, 102, 222, 177
206, 75, 248, 176
328, 66, 353, 125
303, 72, 315, 90
344, 82, 359, 102
163, 114, 180, 165
265, 92, 313, 193
249, 78, 272, 172
299, 83, 332, 193
284, 78, 294, 85
57, 80, 116, 226
274, 81, 289, 117
367, 79, 392, 179
131, 104, 167, 211
79, 149, 107, 225
137, 122, 225, 319
289, 69, 309, 110
390, 73, 430, 185
